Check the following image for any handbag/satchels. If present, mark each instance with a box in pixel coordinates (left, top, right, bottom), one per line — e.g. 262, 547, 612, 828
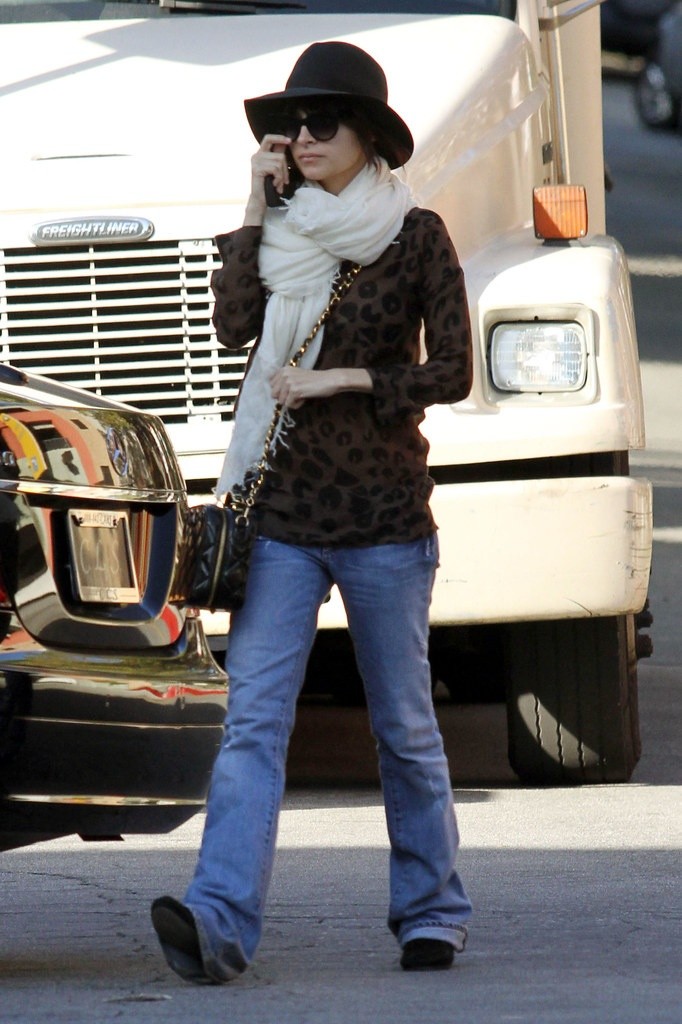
166, 503, 256, 610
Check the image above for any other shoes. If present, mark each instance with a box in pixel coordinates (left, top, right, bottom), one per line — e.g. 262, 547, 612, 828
151, 894, 201, 957
399, 938, 454, 971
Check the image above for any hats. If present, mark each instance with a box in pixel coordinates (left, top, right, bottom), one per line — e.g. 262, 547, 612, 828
243, 40, 414, 171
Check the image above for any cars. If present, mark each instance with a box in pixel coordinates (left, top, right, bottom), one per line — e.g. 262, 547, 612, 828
602, 1, 681, 130
1, 363, 230, 854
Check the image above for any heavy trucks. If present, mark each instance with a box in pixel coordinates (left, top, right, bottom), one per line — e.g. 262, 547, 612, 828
0, 1, 654, 789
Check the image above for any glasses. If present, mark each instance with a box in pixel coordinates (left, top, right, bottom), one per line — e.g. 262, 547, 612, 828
285, 109, 347, 142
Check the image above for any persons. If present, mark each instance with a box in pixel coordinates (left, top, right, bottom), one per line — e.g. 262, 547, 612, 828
150, 41, 472, 985
